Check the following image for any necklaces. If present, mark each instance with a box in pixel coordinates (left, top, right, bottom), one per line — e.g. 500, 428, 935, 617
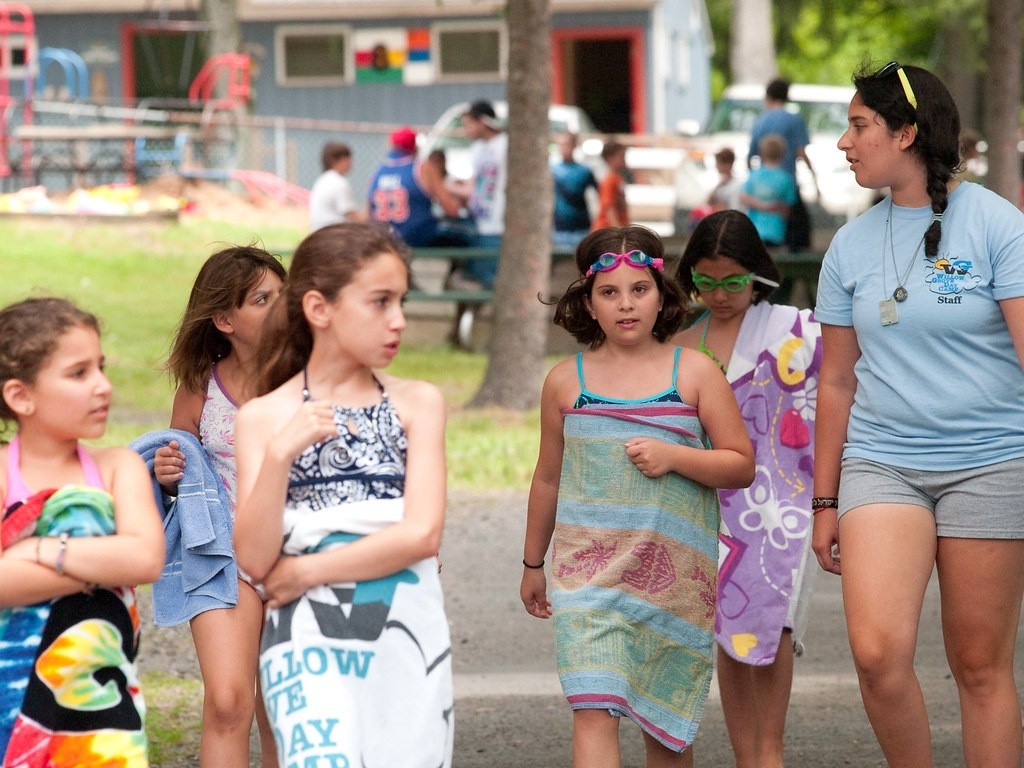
878, 191, 924, 326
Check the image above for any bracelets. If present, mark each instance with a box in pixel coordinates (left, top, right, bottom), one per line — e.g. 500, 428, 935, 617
522, 559, 545, 568
36, 537, 44, 565
55, 533, 69, 573
812, 497, 838, 515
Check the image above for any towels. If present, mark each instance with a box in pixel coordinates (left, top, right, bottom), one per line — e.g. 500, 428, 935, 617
711, 301, 823, 667
0, 486, 149, 768
259, 498, 454, 768
549, 402, 720, 754
128, 425, 239, 628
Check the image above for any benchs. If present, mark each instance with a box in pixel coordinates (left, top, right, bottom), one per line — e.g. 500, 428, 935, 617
401, 289, 565, 304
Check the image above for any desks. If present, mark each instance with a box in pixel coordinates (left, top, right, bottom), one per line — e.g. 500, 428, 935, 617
273, 249, 575, 354
770, 253, 823, 309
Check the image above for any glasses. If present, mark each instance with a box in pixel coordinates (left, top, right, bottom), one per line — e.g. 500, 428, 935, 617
585, 250, 665, 277
875, 60, 919, 136
692, 272, 755, 293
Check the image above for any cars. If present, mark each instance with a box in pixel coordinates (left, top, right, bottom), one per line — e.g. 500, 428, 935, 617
410, 98, 594, 213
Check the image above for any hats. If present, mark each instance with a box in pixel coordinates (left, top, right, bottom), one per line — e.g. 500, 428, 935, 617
390, 128, 416, 154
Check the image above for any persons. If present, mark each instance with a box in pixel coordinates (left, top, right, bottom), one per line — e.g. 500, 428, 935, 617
368, 100, 508, 289
666, 210, 823, 768
309, 142, 367, 229
552, 132, 600, 231
592, 143, 628, 230
0, 298, 167, 768
233, 224, 454, 768
810, 63, 1024, 768
155, 247, 288, 768
709, 77, 821, 251
519, 226, 756, 768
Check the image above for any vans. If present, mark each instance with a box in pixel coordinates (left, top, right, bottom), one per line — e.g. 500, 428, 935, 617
671, 83, 893, 302
